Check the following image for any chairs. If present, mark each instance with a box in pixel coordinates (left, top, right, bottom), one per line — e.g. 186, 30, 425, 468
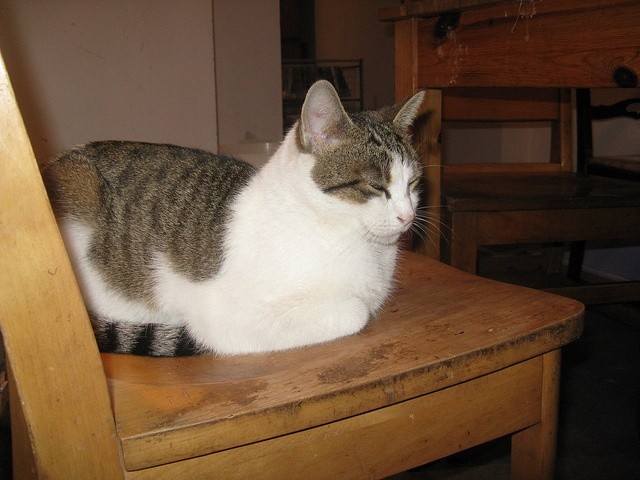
443, 86, 639, 306
0, 48, 587, 479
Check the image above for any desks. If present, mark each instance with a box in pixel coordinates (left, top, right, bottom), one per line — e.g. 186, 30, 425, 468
378, 1, 638, 264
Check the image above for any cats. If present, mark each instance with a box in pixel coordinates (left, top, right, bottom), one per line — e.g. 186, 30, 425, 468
38, 79, 455, 358
